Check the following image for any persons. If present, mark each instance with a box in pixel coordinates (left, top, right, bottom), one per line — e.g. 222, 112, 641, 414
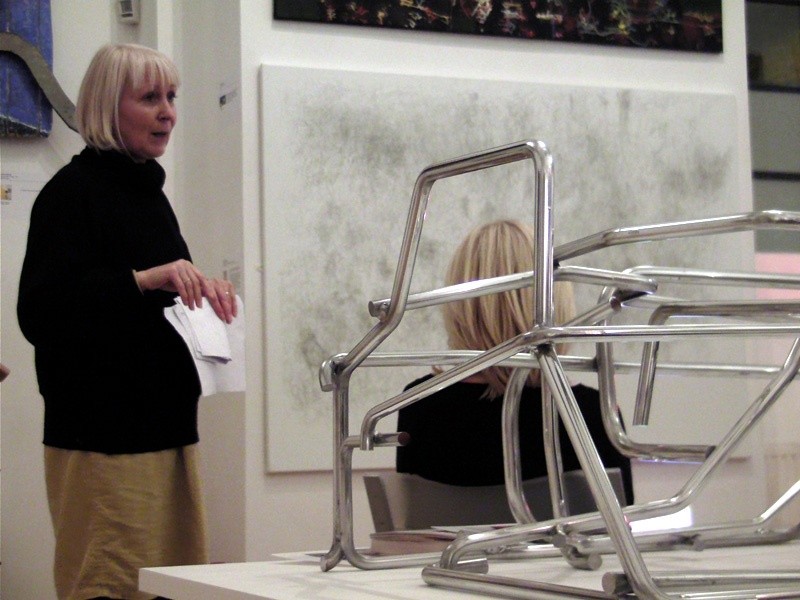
17, 45, 239, 600
396, 221, 636, 508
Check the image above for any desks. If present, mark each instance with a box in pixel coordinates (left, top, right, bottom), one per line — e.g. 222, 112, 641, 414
140, 541, 800, 600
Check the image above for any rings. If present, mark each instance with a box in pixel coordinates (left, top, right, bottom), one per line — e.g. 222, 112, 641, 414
184, 278, 190, 282
225, 292, 229, 294
